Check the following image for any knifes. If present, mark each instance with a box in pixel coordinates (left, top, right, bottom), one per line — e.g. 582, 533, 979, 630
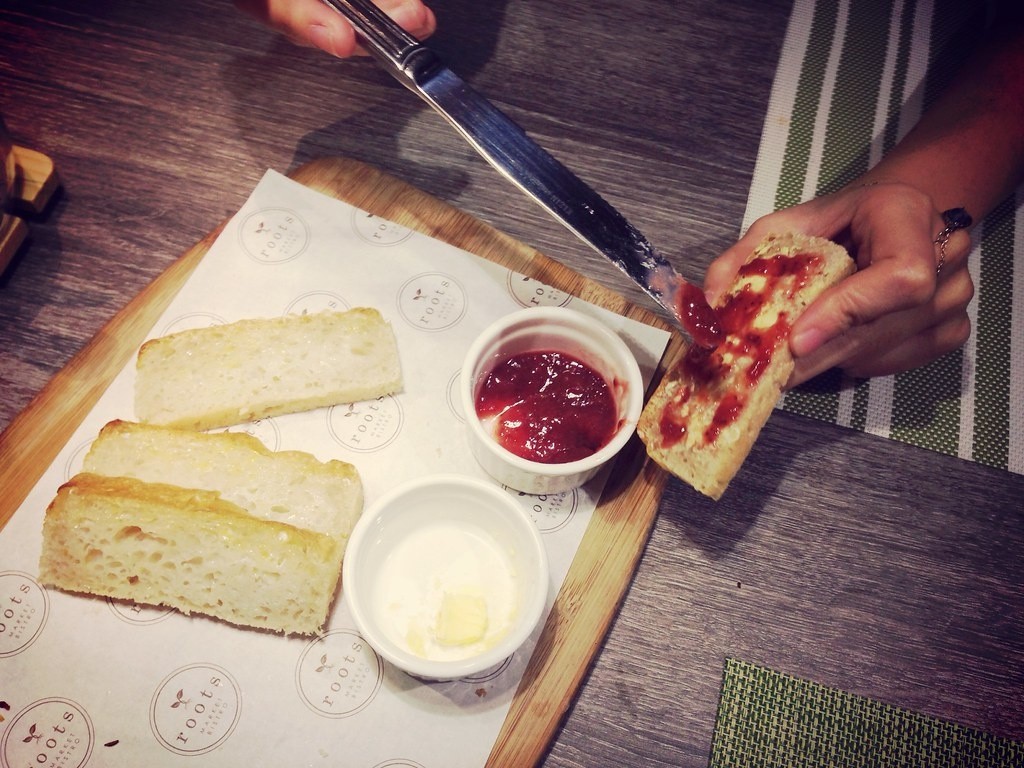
320, 0, 716, 347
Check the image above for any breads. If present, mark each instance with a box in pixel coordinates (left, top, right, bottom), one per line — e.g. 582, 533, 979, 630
82, 419, 364, 553
36, 473, 343, 638
636, 230, 859, 500
133, 308, 403, 430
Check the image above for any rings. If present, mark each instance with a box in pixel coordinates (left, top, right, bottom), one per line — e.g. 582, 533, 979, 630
934, 207, 973, 277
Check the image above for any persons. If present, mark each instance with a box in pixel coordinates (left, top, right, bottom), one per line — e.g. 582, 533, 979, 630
267, 0, 1024, 392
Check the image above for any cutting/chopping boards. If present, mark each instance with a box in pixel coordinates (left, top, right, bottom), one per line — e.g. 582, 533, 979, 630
0, 154, 681, 768
0, 142, 58, 273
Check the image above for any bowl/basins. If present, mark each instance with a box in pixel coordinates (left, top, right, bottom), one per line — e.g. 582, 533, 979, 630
461, 306, 645, 495
342, 476, 550, 678
